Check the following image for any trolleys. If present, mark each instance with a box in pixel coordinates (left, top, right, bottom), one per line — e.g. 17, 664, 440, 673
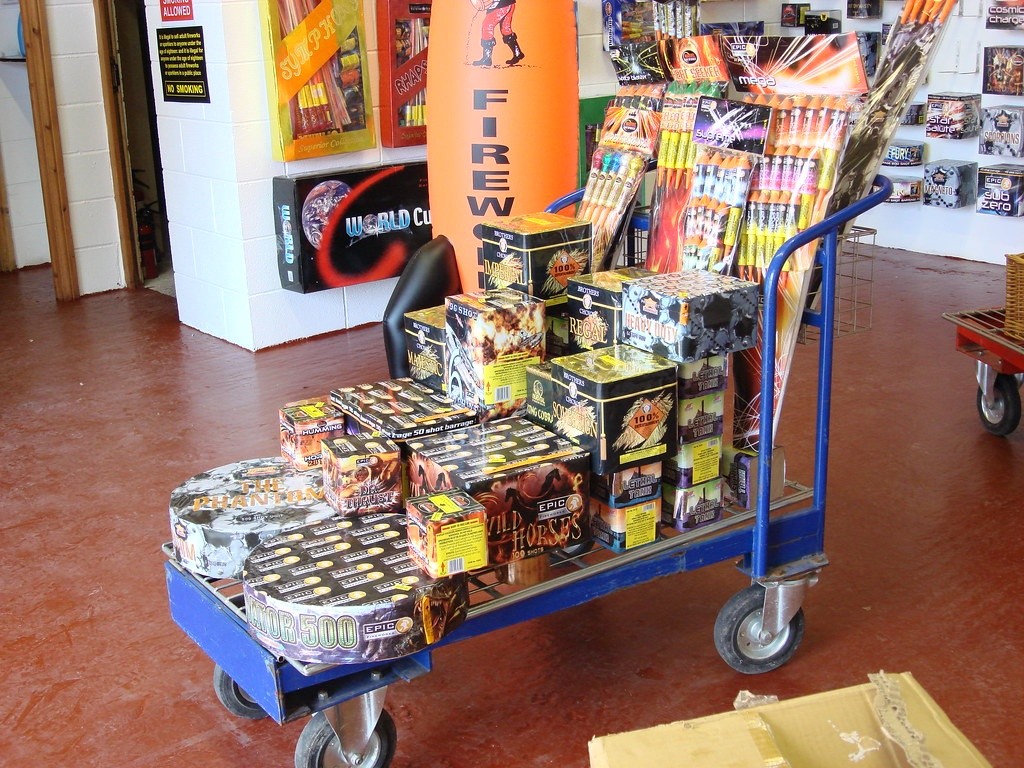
940, 304, 1024, 435
157, 173, 894, 768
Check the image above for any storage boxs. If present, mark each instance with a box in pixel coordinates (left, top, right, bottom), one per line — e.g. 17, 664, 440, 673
869, 45, 1024, 217
587, 669, 993, 768
169, 157, 787, 666
